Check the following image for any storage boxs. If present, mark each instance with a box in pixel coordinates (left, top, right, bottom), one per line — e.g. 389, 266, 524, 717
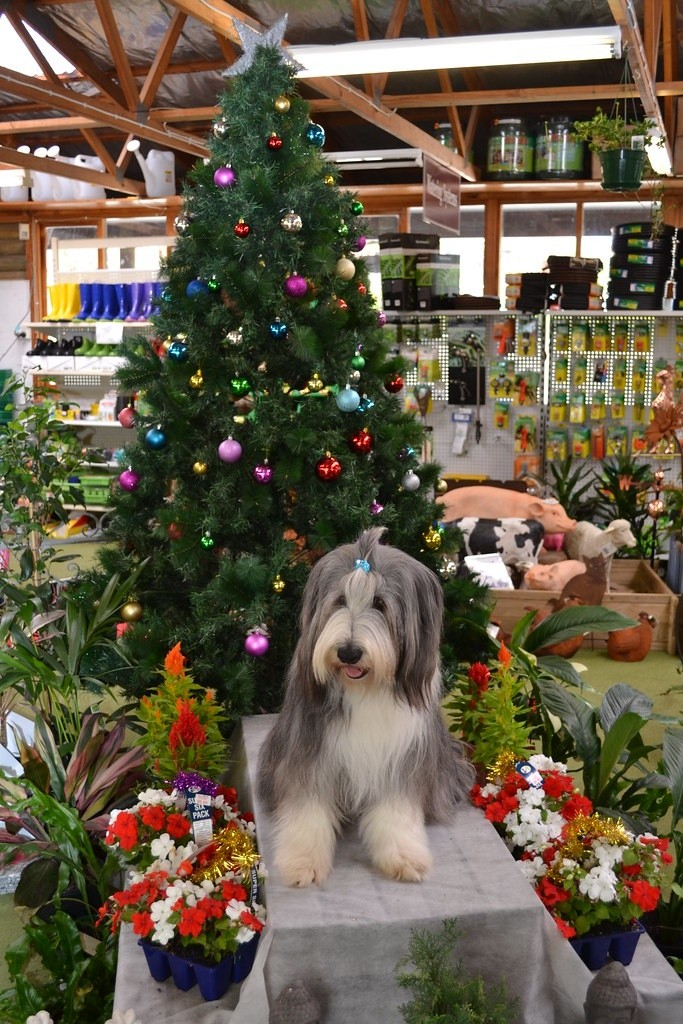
379, 233, 459, 312
79, 470, 124, 504
437, 557, 683, 655
49, 468, 103, 503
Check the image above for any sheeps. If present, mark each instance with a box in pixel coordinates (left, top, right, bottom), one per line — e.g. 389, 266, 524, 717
563, 519, 637, 593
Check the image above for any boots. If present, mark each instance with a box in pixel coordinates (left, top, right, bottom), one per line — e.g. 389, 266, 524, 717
43, 282, 161, 321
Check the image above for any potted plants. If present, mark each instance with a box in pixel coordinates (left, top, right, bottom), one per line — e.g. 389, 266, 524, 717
570, 101, 674, 242
656, 489, 683, 594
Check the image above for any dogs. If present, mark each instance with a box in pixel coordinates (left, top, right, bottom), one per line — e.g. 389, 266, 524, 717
250, 525, 476, 889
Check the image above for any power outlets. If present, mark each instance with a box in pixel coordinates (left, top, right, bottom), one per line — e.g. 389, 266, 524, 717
18, 323, 31, 341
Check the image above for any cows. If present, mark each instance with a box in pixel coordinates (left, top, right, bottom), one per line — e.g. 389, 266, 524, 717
438, 516, 544, 591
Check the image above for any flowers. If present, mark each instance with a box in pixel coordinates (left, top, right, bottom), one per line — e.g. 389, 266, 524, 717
94, 642, 270, 963
469, 639, 673, 939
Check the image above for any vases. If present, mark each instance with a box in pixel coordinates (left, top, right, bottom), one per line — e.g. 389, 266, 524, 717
568, 918, 646, 971
136, 924, 260, 1001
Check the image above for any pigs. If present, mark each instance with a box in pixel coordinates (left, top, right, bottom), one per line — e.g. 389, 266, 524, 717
521, 557, 586, 591
435, 485, 576, 556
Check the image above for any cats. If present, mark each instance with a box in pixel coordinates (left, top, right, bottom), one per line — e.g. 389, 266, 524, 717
559, 552, 606, 608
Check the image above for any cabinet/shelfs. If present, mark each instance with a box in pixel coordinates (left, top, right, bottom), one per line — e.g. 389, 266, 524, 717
21, 235, 183, 542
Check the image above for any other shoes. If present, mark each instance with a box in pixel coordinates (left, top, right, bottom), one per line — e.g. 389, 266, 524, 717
26, 336, 122, 356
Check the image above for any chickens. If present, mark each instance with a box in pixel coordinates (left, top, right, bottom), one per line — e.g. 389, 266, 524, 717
606, 611, 657, 662
488, 595, 594, 660
